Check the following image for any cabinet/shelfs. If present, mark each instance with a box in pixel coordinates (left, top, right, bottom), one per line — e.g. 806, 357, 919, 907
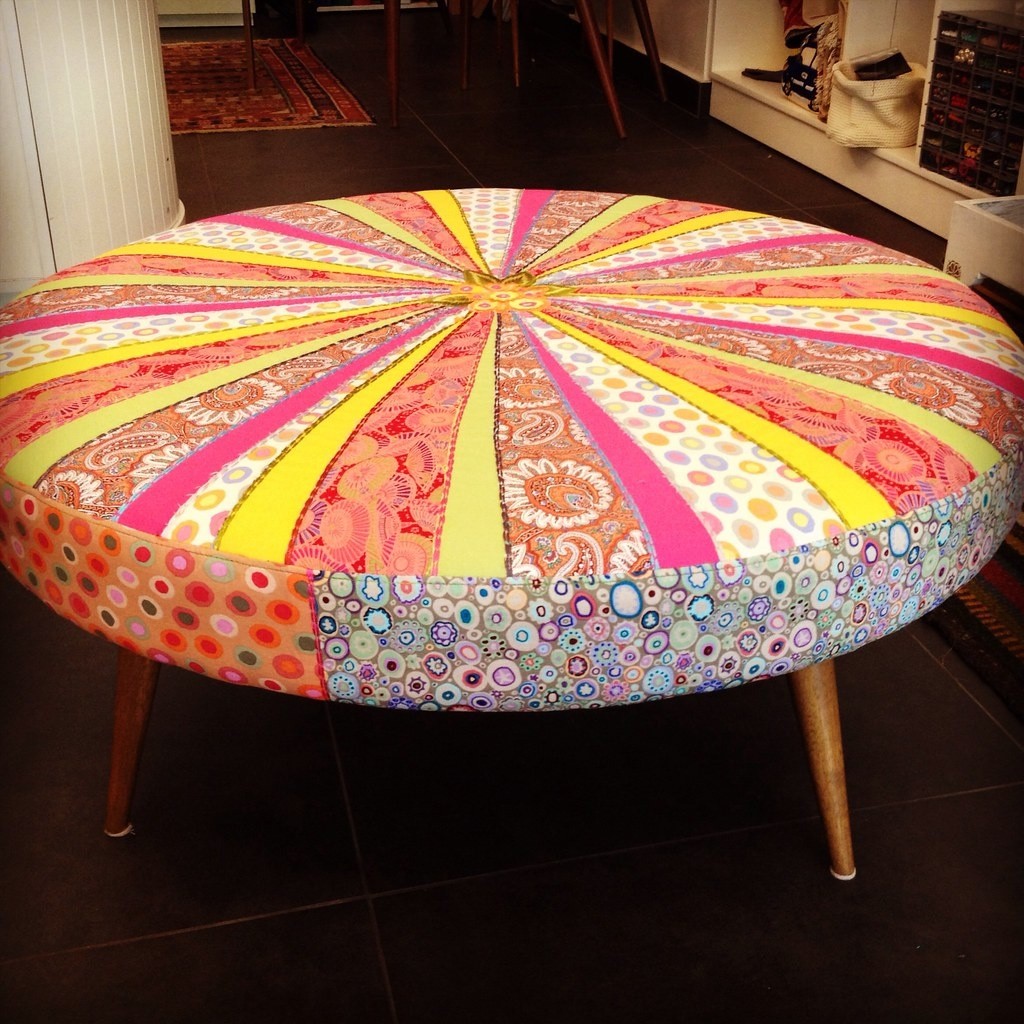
567, 0, 1023, 307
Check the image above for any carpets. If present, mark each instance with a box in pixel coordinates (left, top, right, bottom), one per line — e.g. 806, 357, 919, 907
161, 37, 376, 136
928, 505, 1024, 734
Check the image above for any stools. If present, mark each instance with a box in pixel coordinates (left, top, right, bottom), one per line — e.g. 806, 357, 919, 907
0, 186, 1024, 881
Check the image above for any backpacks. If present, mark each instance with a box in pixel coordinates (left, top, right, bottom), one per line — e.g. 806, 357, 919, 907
741, 22, 825, 116
